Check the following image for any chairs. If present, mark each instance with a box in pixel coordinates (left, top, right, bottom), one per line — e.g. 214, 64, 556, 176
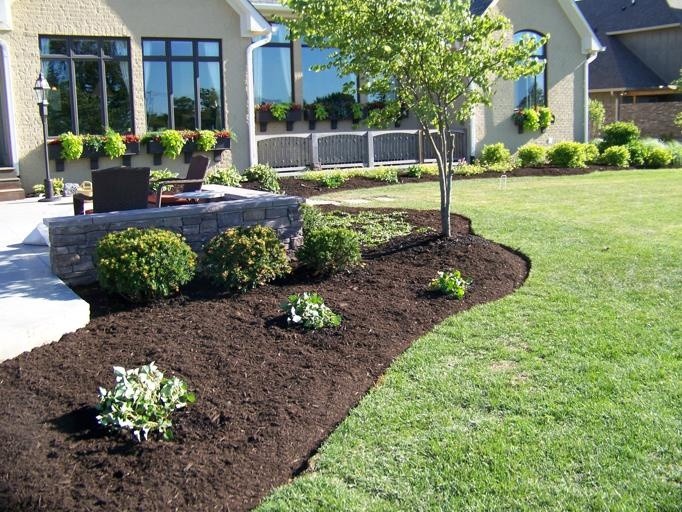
73, 154, 212, 216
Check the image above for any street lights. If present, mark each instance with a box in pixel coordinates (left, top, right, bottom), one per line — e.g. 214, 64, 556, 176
33, 72, 59, 203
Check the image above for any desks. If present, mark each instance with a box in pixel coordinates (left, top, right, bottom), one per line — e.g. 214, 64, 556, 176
175, 190, 226, 202
